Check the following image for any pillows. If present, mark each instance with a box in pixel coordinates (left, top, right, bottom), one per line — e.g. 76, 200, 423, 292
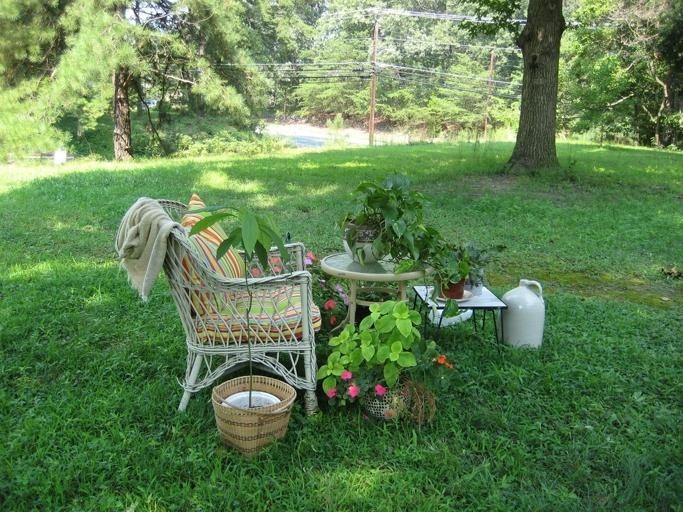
181, 194, 245, 314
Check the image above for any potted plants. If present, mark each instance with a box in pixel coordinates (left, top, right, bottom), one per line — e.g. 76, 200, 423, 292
339, 168, 442, 269
423, 234, 481, 298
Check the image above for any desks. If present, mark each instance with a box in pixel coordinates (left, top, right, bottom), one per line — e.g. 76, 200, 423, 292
313, 251, 435, 328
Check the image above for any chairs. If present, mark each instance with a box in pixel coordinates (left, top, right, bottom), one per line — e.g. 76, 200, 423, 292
150, 195, 325, 421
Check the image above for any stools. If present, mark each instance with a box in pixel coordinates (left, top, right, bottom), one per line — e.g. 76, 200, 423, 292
410, 284, 508, 366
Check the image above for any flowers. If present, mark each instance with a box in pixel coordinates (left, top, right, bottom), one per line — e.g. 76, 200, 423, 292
319, 294, 442, 404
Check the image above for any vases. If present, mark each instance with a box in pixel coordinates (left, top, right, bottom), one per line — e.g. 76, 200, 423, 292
361, 382, 414, 418
